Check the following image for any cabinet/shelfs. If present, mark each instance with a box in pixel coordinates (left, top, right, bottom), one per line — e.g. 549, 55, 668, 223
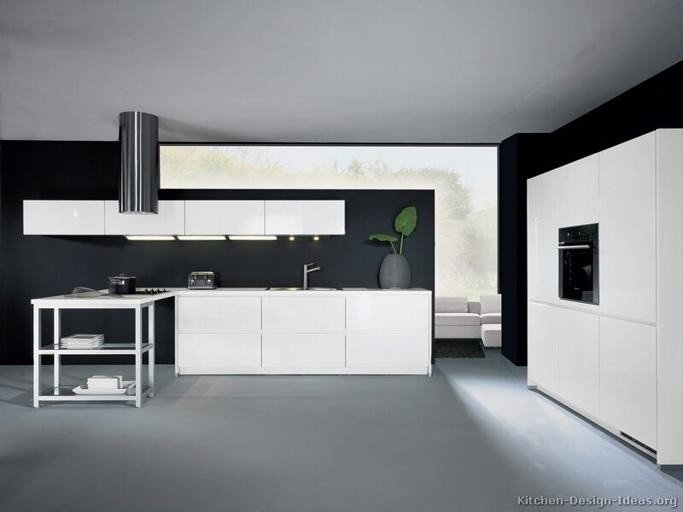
348, 296, 429, 378
264, 297, 346, 374
104, 200, 184, 235
597, 132, 657, 326
184, 200, 265, 238
527, 173, 558, 304
176, 296, 262, 377
265, 201, 345, 236
22, 199, 103, 236
525, 301, 657, 453
30, 298, 155, 409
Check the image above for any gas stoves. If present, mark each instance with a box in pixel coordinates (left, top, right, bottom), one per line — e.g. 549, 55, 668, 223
129, 288, 172, 296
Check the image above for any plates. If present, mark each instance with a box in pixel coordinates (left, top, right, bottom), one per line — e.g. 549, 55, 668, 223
87, 374, 123, 390
59, 334, 104, 349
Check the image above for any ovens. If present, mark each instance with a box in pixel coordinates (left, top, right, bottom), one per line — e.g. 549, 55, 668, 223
558, 224, 599, 305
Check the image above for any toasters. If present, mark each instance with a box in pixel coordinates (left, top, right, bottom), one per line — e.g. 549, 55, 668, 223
187, 271, 218, 290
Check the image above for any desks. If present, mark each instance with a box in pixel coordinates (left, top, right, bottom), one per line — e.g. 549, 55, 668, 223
482, 324, 502, 349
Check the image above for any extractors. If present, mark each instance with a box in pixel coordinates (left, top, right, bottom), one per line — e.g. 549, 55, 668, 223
118, 113, 159, 216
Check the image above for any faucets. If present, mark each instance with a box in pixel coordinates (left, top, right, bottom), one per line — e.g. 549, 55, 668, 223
303, 263, 321, 289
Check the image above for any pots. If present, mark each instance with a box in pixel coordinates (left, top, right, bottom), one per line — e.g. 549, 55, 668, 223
107, 273, 136, 294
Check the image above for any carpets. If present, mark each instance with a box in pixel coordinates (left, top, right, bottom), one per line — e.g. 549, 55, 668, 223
434, 338, 487, 360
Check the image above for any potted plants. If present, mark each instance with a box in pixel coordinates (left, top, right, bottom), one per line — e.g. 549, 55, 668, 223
368, 205, 419, 289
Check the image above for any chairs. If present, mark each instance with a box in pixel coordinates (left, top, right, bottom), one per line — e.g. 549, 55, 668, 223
480, 294, 502, 322
434, 295, 480, 340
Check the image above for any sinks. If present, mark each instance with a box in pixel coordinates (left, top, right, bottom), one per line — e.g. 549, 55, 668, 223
308, 286, 343, 290
265, 287, 304, 290
